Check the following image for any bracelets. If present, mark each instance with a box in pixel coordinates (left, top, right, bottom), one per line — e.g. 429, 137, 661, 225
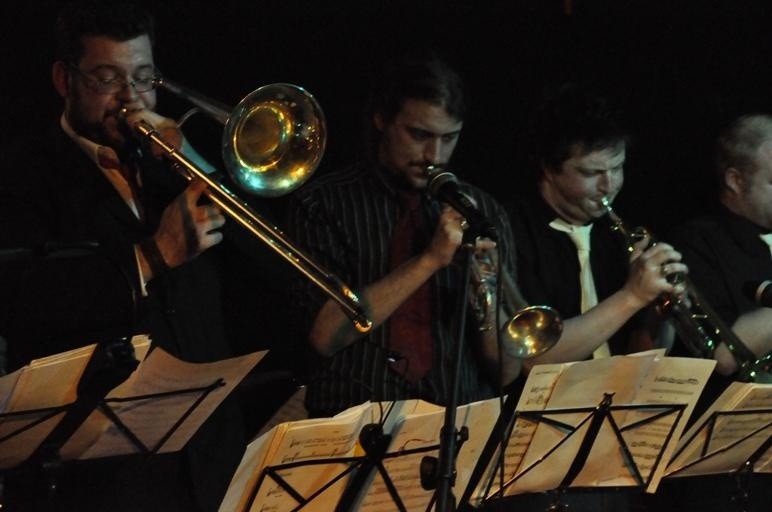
137, 237, 172, 278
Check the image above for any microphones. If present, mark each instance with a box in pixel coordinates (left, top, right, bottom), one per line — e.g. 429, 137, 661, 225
427, 169, 490, 234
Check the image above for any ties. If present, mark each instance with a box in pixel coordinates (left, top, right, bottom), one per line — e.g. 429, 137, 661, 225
549, 216, 611, 358
98, 154, 148, 222
388, 189, 431, 388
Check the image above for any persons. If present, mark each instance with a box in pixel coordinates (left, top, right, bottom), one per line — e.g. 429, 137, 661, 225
655, 114, 770, 512
500, 89, 689, 511
284, 53, 527, 428
0, 1, 296, 512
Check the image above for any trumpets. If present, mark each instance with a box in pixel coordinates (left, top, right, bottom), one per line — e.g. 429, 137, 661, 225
597, 197, 772, 383
423, 165, 563, 360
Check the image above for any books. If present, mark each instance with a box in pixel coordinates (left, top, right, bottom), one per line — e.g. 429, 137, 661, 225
1, 335, 772, 511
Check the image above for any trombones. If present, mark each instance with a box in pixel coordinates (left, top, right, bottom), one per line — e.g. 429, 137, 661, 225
114, 78, 373, 334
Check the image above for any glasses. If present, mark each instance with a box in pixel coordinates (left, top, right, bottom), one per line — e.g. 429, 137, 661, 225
66, 61, 165, 94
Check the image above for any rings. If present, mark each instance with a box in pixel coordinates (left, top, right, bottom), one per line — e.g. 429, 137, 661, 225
660, 263, 667, 280
458, 217, 469, 230
